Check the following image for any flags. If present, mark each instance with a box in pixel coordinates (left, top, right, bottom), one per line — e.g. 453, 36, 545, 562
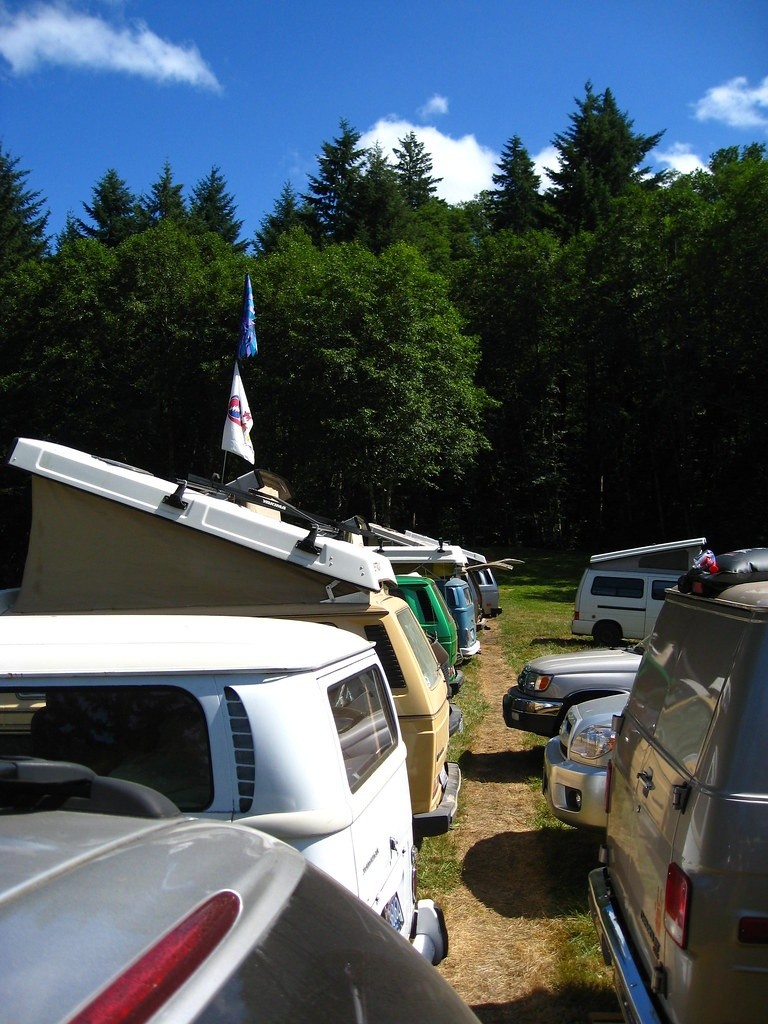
221, 360, 255, 464
239, 272, 257, 358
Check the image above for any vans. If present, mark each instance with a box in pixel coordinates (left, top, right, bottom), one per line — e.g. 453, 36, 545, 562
586, 576, 767, 1024
1, 430, 526, 1024
570, 538, 710, 648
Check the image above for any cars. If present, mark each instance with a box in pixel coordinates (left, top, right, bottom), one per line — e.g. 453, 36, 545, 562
502, 629, 655, 735
542, 691, 632, 832
